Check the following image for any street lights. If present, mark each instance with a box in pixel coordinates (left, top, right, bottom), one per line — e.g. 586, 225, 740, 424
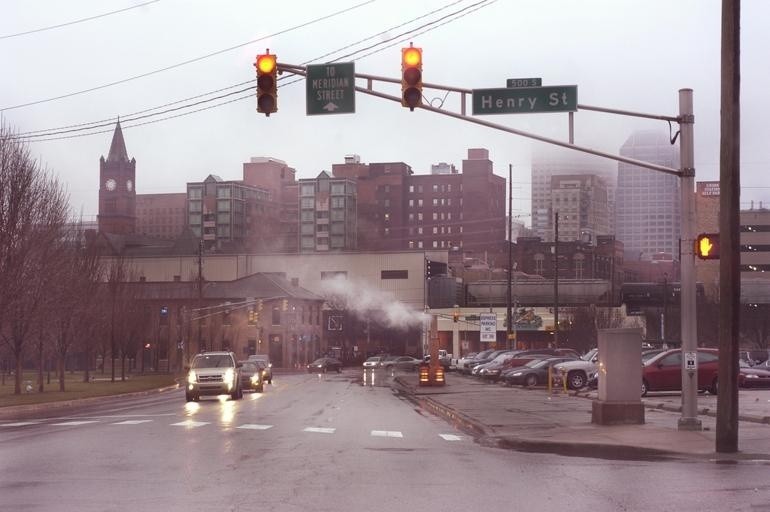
465, 256, 494, 352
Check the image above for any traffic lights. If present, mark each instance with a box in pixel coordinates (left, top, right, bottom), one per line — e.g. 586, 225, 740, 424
400, 46, 423, 109
255, 53, 278, 117
258, 300, 263, 312
253, 312, 259, 323
249, 310, 254, 321
454, 310, 459, 323
282, 299, 289, 312
696, 230, 721, 262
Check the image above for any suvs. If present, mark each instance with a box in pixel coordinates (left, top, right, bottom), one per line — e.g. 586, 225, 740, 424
184, 347, 244, 402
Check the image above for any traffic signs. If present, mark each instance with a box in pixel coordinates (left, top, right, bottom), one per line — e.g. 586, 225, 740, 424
304, 61, 356, 117
471, 77, 578, 116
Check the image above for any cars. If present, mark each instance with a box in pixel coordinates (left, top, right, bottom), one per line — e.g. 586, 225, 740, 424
305, 357, 344, 375
237, 352, 273, 394
